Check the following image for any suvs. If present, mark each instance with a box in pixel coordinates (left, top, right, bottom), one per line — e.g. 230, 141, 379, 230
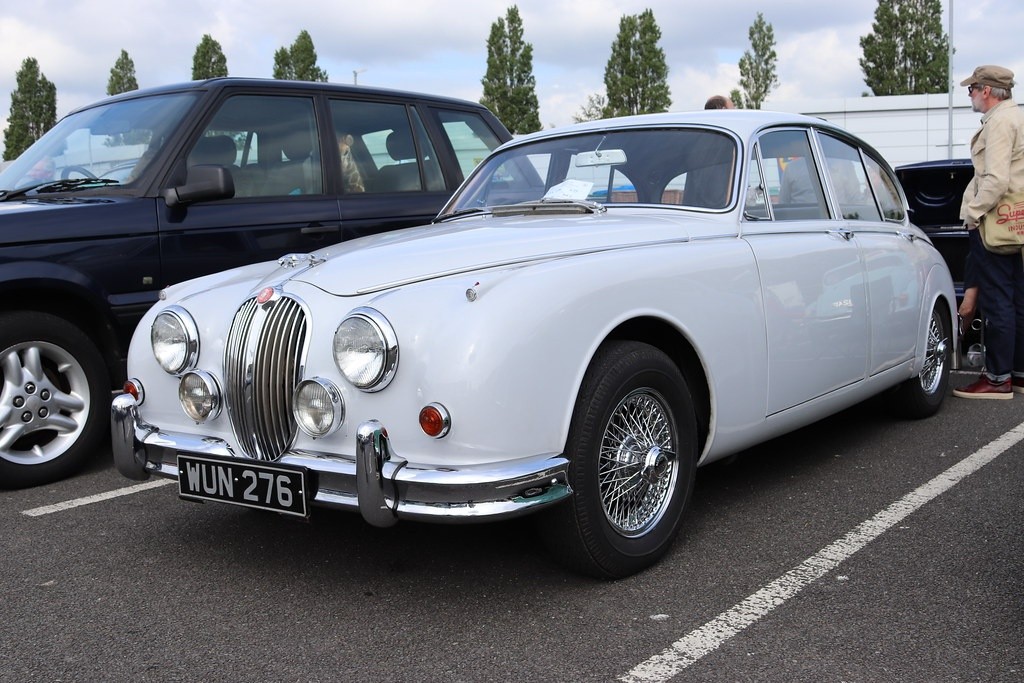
0, 72, 549, 495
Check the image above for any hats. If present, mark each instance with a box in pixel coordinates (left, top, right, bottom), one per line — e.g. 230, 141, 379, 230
960, 65, 1015, 90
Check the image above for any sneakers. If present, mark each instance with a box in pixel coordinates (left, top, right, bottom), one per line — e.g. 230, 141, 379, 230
1012, 373, 1024, 394
953, 374, 1014, 400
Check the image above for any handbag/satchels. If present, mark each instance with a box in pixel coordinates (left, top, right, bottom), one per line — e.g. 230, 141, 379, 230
978, 191, 1024, 253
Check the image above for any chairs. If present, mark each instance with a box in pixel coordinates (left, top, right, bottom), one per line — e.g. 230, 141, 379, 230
245, 115, 323, 197
381, 128, 444, 193
188, 134, 249, 197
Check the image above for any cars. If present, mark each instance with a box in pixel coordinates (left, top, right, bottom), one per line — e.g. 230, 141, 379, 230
105, 106, 961, 581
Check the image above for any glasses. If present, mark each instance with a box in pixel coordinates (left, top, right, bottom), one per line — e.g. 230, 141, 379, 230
968, 86, 981, 93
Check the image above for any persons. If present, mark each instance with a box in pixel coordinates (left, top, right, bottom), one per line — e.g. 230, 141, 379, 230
777, 117, 858, 206
20, 157, 57, 181
952, 65, 1024, 400
679, 95, 735, 208
